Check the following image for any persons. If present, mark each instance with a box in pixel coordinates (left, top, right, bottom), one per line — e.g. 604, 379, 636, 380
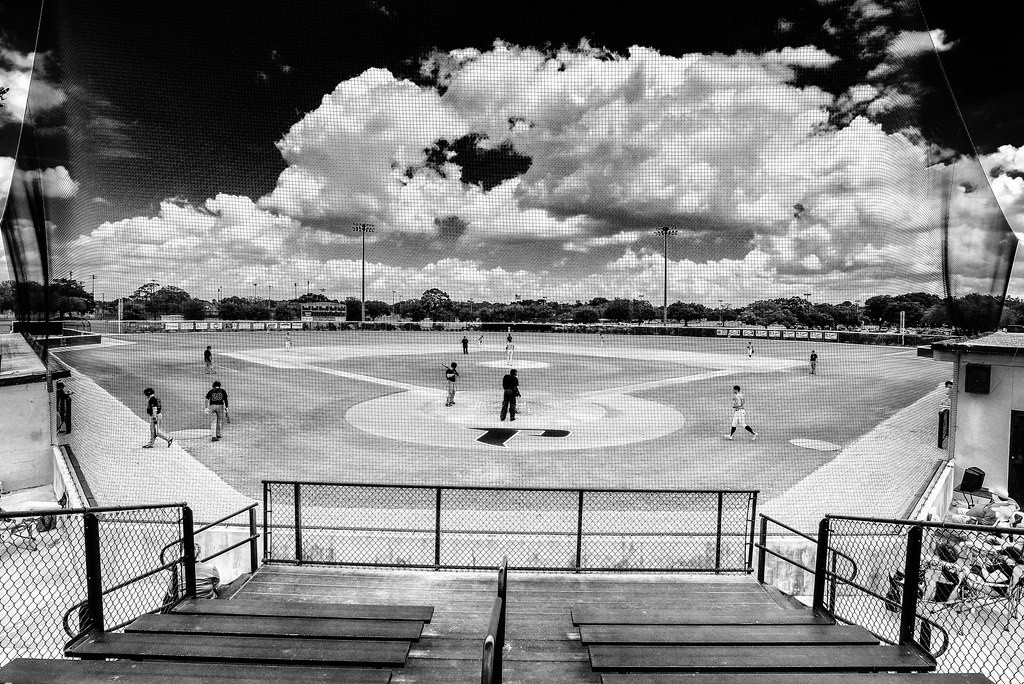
898, 546, 1021, 606
57, 382, 66, 434
285, 333, 291, 350
939, 381, 953, 411
500, 368, 521, 420
951, 487, 1020, 547
724, 385, 758, 440
204, 345, 216, 374
143, 387, 173, 448
446, 363, 459, 406
810, 351, 818, 374
204, 381, 229, 441
505, 332, 514, 366
462, 336, 469, 354
747, 342, 753, 359
600, 333, 604, 348
479, 335, 484, 344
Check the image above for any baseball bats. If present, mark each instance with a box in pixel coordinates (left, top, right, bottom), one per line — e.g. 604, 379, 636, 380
225, 407, 230, 424
442, 363, 459, 375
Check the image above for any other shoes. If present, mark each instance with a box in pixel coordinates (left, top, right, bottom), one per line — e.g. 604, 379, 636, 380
217, 435, 221, 438
450, 401, 456, 404
446, 403, 452, 406
212, 437, 218, 441
753, 433, 758, 440
724, 435, 733, 439
168, 438, 173, 447
143, 445, 153, 448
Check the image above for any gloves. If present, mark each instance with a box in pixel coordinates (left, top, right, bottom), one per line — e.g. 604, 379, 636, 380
153, 417, 158, 425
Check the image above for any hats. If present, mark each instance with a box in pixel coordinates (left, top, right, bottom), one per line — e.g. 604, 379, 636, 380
945, 381, 953, 385
144, 388, 154, 395
213, 381, 221, 387
451, 363, 457, 368
510, 369, 517, 376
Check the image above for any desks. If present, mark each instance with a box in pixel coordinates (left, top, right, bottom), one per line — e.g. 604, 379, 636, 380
954, 484, 994, 508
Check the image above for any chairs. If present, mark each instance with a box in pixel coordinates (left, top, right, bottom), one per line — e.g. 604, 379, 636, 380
966, 498, 1020, 526
